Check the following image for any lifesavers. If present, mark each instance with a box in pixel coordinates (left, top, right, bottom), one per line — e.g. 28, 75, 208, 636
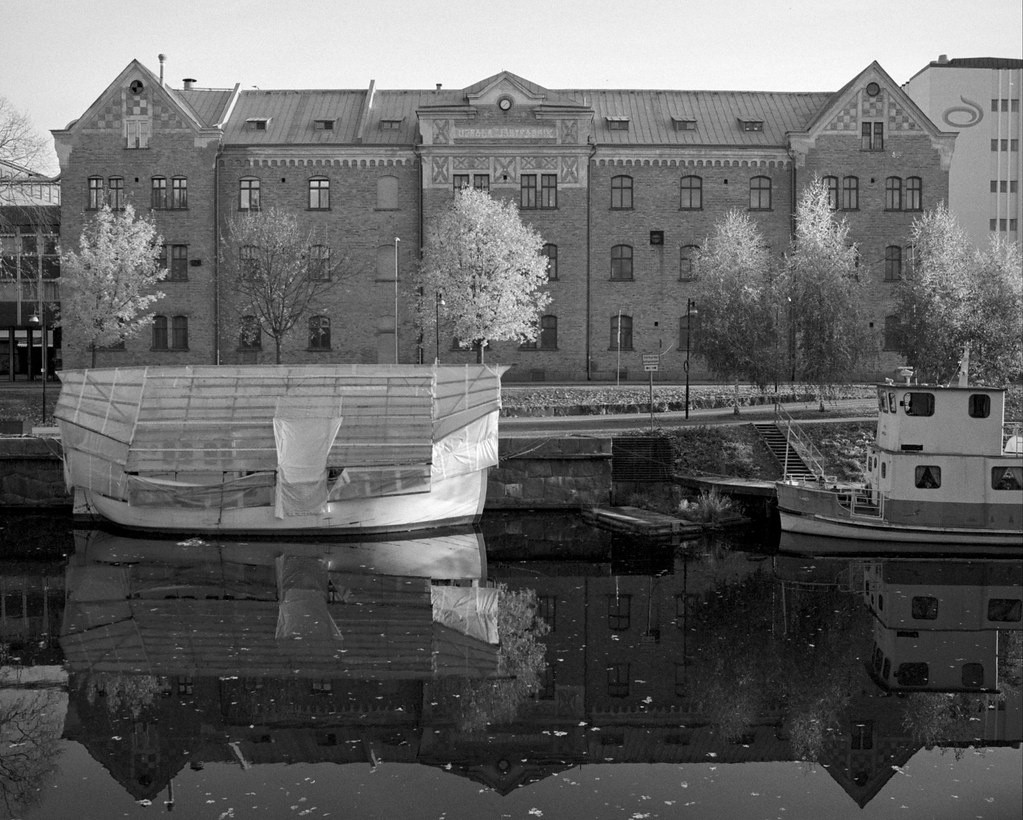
683, 359, 690, 374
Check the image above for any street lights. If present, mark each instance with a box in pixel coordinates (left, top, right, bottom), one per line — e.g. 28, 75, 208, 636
686, 300, 700, 420
29, 310, 45, 423
435, 293, 446, 364
394, 237, 400, 363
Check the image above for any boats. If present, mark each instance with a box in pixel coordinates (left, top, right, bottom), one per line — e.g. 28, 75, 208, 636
777, 336, 1023, 555
773, 553, 1022, 694
82, 442, 488, 531
